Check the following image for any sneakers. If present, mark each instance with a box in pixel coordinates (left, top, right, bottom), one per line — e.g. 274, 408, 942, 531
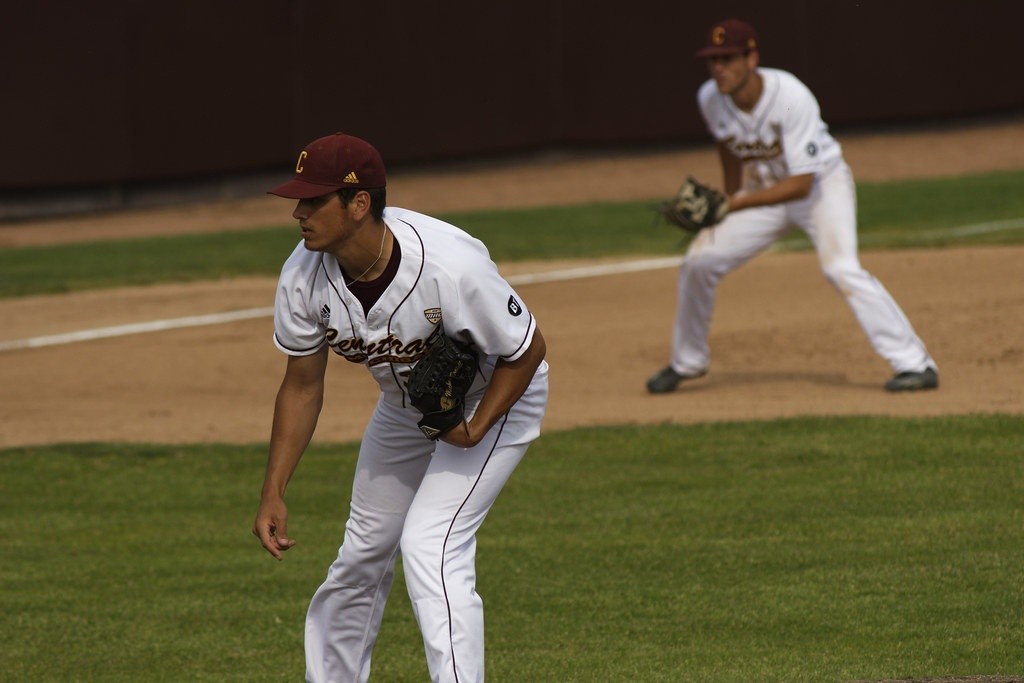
884, 367, 939, 391
646, 366, 707, 392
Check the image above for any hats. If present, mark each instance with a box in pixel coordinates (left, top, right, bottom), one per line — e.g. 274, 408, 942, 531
265, 132, 386, 198
696, 18, 759, 56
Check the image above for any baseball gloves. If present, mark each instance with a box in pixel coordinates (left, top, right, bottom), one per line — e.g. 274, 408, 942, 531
664, 175, 731, 233
406, 336, 479, 439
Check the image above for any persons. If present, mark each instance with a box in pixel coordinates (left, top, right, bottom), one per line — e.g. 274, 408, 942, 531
647, 18, 939, 394
251, 132, 550, 683
